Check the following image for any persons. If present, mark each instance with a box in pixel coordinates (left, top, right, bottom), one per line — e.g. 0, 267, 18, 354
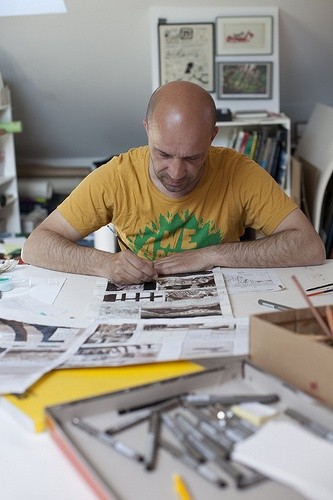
21, 80, 327, 286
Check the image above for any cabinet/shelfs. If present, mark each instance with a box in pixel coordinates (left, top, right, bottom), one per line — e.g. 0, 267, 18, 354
214, 114, 291, 204
0, 80, 22, 235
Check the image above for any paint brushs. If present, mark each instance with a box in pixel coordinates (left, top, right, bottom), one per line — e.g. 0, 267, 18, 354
107, 220, 165, 289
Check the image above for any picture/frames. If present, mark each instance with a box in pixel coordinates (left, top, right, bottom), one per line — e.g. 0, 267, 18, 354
217, 14, 274, 57
157, 22, 216, 94
217, 61, 273, 99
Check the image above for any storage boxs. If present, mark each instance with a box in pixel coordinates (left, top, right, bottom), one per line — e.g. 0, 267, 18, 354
246, 305, 333, 407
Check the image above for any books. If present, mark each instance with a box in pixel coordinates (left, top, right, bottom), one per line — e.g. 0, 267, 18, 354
229, 127, 288, 191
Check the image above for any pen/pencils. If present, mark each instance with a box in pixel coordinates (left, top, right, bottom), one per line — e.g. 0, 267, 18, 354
257, 297, 301, 314
298, 282, 333, 297
69, 383, 332, 499
